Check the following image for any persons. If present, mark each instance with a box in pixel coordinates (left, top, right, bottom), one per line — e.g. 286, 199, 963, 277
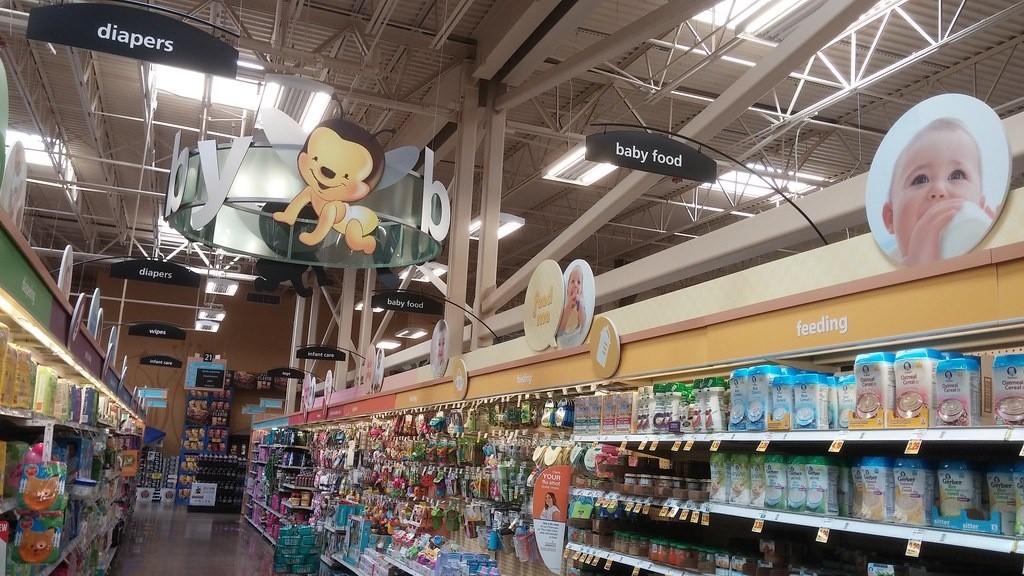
882, 116, 1003, 268
558, 265, 587, 334
540, 491, 562, 522
433, 320, 447, 377
373, 349, 382, 392
8, 142, 27, 230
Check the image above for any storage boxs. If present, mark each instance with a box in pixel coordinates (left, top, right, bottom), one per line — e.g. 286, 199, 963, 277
324, 500, 396, 576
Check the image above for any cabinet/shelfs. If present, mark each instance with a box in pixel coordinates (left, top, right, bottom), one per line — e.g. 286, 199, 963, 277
566, 187, 1024, 576
0, 204, 147, 576
242, 405, 319, 546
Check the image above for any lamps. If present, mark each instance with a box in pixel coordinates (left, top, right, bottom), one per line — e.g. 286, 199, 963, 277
251, 73, 335, 146
375, 339, 402, 350
195, 309, 227, 333
734, 0, 829, 48
541, 141, 619, 187
354, 300, 384, 313
204, 278, 239, 297
394, 327, 429, 340
468, 213, 525, 240
398, 261, 448, 282
